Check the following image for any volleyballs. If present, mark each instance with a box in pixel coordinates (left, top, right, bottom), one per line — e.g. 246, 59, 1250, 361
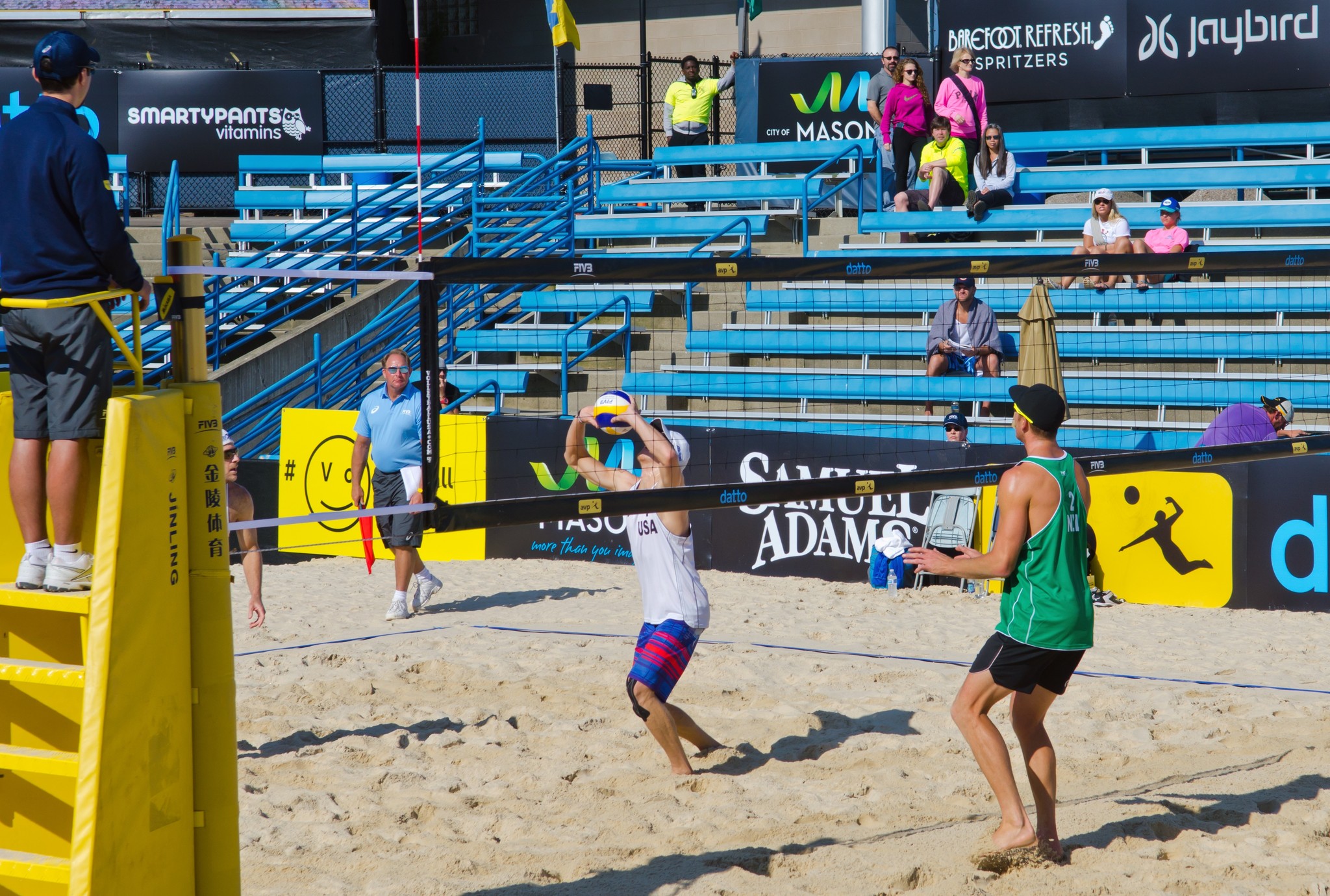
593, 390, 633, 435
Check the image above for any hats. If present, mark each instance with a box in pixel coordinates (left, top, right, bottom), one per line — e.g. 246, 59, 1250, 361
650, 418, 690, 474
1156, 197, 1180, 212
439, 356, 446, 369
1008, 383, 1066, 431
222, 428, 235, 446
1093, 188, 1114, 201
1260, 395, 1295, 422
33, 31, 102, 82
943, 413, 968, 429
952, 278, 975, 288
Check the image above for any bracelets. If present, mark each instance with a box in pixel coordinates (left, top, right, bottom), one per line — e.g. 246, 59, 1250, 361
418, 488, 423, 493
578, 409, 585, 424
439, 396, 446, 398
974, 348, 980, 356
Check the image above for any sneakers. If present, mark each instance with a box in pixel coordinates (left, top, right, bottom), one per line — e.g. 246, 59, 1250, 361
1090, 586, 1127, 607
410, 573, 443, 611
385, 598, 410, 620
1083, 277, 1097, 289
1044, 278, 1066, 290
16, 551, 93, 592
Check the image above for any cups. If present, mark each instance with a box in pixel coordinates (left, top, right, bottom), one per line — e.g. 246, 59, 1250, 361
975, 579, 984, 598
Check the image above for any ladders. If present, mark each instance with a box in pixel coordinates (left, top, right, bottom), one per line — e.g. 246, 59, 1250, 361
0, 386, 197, 895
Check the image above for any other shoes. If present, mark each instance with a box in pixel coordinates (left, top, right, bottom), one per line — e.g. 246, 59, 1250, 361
917, 199, 932, 211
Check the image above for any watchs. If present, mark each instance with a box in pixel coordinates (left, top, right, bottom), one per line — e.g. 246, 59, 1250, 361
440, 398, 449, 404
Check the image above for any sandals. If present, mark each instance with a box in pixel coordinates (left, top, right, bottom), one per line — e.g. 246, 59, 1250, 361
966, 190, 986, 221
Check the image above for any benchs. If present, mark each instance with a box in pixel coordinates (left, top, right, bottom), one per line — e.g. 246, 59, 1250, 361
108, 123, 1330, 453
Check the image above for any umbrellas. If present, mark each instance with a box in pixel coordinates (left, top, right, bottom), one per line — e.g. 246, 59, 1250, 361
1011, 276, 1071, 429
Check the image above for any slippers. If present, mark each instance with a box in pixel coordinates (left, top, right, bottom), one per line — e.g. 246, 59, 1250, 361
1092, 282, 1110, 290
1136, 283, 1149, 290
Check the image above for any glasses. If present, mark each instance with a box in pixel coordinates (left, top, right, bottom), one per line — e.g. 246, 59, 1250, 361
985, 135, 1000, 140
438, 368, 448, 374
224, 447, 239, 461
903, 69, 918, 75
1284, 422, 1290, 427
385, 365, 409, 374
1094, 199, 1109, 204
945, 426, 963, 432
883, 56, 899, 60
959, 59, 976, 64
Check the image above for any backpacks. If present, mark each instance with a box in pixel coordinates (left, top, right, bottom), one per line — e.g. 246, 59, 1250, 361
869, 544, 911, 590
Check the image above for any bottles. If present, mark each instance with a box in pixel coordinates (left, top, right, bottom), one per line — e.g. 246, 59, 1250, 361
967, 578, 976, 594
888, 569, 897, 597
950, 401, 959, 414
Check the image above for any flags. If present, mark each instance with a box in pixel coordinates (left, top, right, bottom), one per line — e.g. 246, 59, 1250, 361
545, 0, 580, 51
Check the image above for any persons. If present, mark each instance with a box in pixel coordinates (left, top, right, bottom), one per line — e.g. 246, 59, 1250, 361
564, 395, 720, 776
1193, 396, 1311, 448
220, 429, 265, 628
866, 45, 1016, 244
925, 278, 1004, 417
411, 357, 461, 415
942, 412, 968, 442
1045, 188, 1189, 290
902, 383, 1092, 861
0, 31, 151, 593
663, 52, 740, 212
352, 349, 442, 620
